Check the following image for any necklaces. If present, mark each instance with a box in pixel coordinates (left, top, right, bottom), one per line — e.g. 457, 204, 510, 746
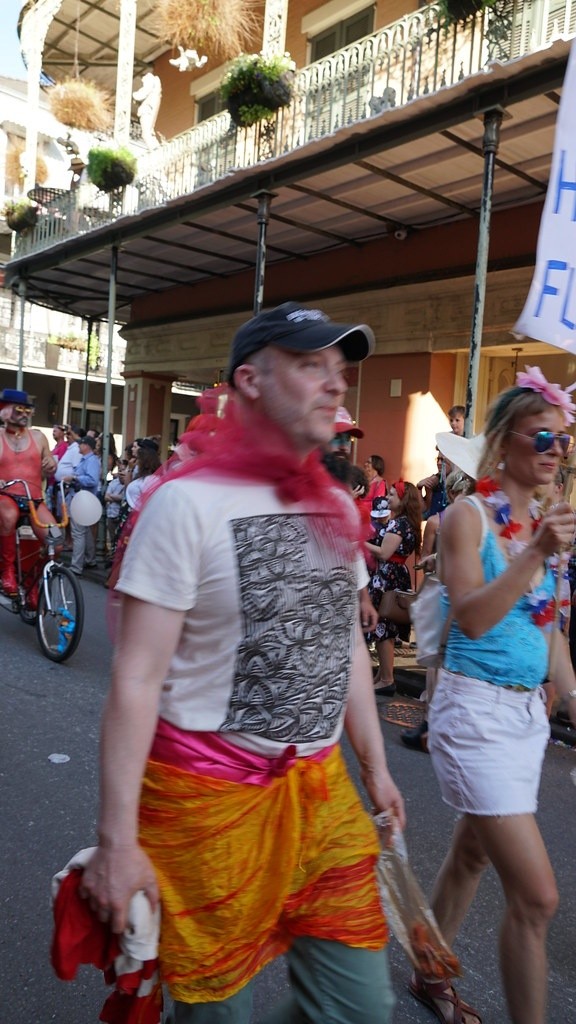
5, 427, 24, 439
480, 476, 570, 632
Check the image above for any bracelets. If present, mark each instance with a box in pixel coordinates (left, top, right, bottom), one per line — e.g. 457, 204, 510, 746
561, 690, 576, 704
126, 469, 133, 472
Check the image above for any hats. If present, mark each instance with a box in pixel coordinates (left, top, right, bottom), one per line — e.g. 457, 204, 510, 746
137, 438, 159, 452
231, 302, 376, 384
334, 407, 364, 439
75, 435, 97, 449
0, 389, 33, 408
370, 495, 391, 518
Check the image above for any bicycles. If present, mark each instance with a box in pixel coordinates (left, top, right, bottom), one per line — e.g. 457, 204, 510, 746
0, 477, 85, 662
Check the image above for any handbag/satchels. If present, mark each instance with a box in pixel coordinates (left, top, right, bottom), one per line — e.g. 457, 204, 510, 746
377, 591, 419, 623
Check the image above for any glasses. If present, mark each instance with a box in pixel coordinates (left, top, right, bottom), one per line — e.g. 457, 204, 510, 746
506, 430, 575, 454
4, 405, 32, 416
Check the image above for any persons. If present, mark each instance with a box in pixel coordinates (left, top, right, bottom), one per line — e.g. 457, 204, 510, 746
401, 372, 576, 1024
322, 402, 576, 756
81, 303, 408, 1024
0, 386, 163, 608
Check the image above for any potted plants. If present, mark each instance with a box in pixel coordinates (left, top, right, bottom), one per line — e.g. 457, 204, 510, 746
76, 144, 136, 208
216, 51, 296, 129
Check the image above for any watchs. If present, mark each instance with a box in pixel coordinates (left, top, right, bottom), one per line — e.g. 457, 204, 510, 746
72, 476, 76, 481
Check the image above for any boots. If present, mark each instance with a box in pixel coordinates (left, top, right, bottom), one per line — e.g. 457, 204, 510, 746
22, 572, 41, 610
0, 530, 18, 593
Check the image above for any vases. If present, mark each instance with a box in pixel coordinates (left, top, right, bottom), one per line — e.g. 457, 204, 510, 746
5, 205, 38, 231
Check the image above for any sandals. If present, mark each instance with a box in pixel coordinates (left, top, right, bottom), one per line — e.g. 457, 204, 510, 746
406, 969, 483, 1024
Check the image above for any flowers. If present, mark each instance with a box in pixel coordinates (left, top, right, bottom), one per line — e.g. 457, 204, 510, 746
514, 364, 576, 427
1, 196, 37, 220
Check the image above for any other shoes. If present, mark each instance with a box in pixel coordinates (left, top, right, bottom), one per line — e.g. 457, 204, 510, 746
400, 720, 429, 751
82, 562, 99, 570
556, 709, 571, 722
393, 637, 402, 649
103, 562, 112, 588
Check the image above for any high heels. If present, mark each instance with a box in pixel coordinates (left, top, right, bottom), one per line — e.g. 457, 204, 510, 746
375, 682, 397, 697
